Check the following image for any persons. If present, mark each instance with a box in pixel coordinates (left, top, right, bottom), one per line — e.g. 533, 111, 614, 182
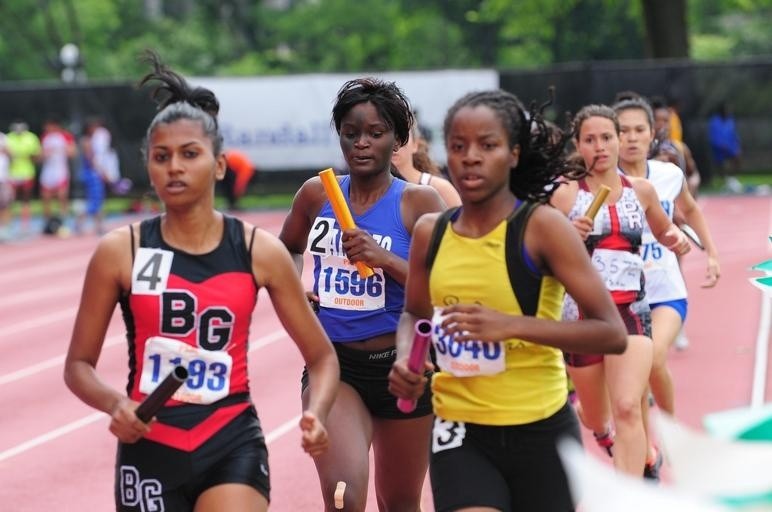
61, 52, 343, 510
276, 79, 447, 511
0, 117, 255, 235
392, 109, 462, 210
389, 87, 628, 511
531, 90, 745, 483
